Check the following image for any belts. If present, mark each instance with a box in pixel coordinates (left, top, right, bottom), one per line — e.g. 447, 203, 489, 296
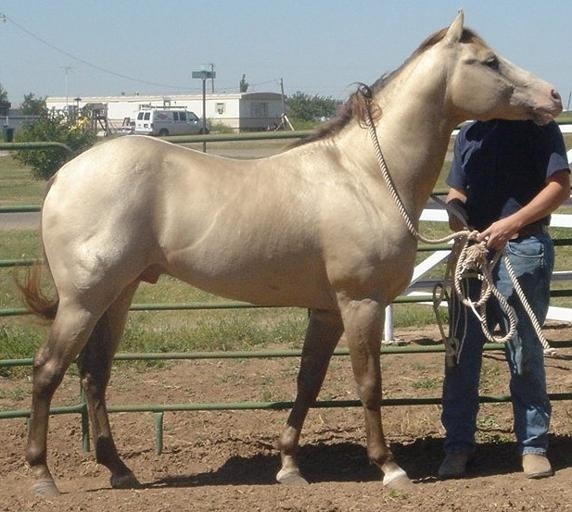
508, 221, 550, 242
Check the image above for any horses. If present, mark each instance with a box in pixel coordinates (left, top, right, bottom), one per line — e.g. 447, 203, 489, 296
12, 7, 563, 505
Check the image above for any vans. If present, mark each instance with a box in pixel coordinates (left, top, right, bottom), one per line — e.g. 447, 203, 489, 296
135, 106, 209, 136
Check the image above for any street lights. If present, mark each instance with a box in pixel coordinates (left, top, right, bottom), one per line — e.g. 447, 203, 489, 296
191, 64, 216, 155
73, 97, 82, 124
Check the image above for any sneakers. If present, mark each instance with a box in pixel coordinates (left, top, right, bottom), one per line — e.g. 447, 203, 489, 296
437, 449, 472, 478
515, 450, 556, 479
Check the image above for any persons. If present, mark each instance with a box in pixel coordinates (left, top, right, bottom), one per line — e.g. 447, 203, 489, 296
437, 119, 571, 478
